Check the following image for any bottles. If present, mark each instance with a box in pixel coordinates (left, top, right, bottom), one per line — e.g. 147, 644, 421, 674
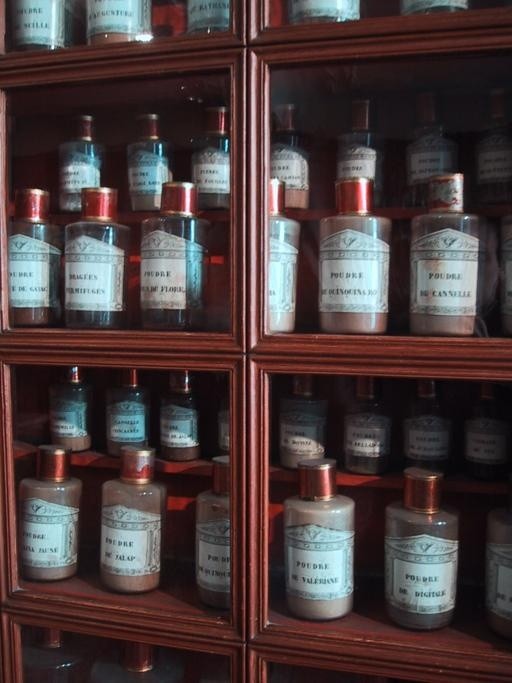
260, 179, 502, 339
13, 623, 191, 683
16, 448, 78, 585
6, 185, 212, 327
58, 112, 237, 208
282, 455, 353, 619
275, 103, 506, 205
190, 456, 244, 610
95, 449, 164, 597
485, 505, 512, 647
384, 465, 461, 632
275, 376, 507, 474
48, 363, 231, 459
9, 0, 241, 55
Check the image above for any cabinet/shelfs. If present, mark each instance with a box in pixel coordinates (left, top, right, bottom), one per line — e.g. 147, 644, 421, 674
0, 1, 510, 683
